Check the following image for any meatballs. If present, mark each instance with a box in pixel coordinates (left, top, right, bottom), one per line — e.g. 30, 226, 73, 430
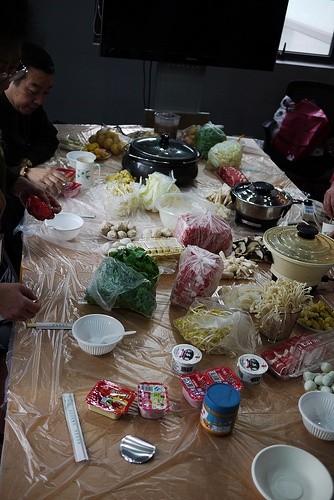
99, 219, 138, 239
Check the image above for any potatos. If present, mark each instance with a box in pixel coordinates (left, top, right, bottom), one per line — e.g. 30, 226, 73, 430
84, 128, 125, 156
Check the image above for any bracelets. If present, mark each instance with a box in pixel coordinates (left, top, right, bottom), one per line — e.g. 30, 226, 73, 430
23, 163, 28, 177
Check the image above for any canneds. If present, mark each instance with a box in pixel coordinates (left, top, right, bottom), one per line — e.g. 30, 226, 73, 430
200, 383, 240, 438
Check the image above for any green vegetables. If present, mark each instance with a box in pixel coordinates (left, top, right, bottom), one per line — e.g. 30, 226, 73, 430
84, 247, 159, 318
194, 126, 228, 159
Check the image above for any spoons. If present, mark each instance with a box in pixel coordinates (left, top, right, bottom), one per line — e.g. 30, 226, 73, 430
93, 331, 137, 345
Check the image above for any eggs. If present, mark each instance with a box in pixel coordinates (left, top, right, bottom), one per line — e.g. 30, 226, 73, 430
302, 362, 334, 395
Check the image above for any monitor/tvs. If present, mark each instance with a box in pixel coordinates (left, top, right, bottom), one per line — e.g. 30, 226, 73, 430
98, 0, 289, 71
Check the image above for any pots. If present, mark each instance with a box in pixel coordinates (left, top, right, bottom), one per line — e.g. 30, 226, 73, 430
122, 132, 200, 186
227, 179, 302, 220
263, 222, 334, 288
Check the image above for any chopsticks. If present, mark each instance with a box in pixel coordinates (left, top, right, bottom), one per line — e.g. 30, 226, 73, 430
26, 322, 36, 327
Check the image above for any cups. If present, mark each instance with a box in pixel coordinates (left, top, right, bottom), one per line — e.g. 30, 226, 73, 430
153, 111, 180, 138
76, 157, 101, 188
320, 221, 334, 238
258, 294, 302, 340
227, 137, 246, 154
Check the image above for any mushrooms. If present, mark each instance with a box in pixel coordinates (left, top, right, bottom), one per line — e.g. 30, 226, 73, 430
233, 235, 273, 262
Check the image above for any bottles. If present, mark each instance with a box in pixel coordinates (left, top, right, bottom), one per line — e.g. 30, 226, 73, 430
200, 383, 239, 436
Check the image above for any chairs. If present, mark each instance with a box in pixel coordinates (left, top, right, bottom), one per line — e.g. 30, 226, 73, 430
264, 80, 334, 184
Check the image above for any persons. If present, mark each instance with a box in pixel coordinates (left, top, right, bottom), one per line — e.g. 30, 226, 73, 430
0, 142, 61, 362
324, 173, 334, 220
0, 46, 72, 198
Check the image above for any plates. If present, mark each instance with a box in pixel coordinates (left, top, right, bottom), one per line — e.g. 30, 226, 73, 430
252, 445, 334, 500
295, 317, 326, 334
98, 230, 140, 240
97, 149, 112, 159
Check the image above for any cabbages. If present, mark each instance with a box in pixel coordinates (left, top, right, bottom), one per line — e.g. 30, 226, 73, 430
208, 140, 242, 170
140, 174, 182, 211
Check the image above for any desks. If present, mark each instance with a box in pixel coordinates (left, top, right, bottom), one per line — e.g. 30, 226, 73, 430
1, 125, 334, 499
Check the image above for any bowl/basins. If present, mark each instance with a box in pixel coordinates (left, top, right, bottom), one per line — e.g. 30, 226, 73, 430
65, 151, 97, 169
153, 192, 209, 229
298, 391, 334, 440
45, 213, 84, 241
56, 168, 83, 199
72, 313, 123, 355
